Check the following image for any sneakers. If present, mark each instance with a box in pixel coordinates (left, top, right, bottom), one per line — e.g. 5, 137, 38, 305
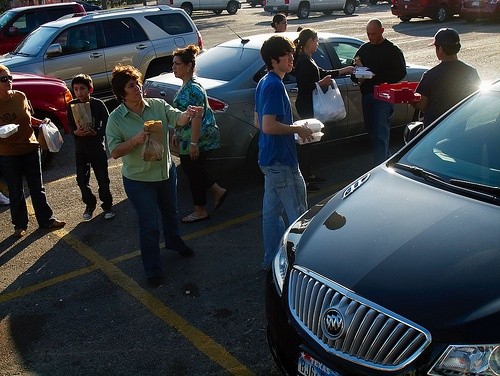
83, 204, 96, 219
305, 175, 328, 184
13, 225, 27, 236
39, 217, 65, 230
105, 210, 115, 219
306, 183, 320, 193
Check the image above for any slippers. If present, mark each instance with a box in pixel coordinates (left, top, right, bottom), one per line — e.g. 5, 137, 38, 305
215, 187, 231, 209
180, 212, 210, 223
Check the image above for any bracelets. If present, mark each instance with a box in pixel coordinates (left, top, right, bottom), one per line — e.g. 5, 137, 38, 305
190, 141, 199, 146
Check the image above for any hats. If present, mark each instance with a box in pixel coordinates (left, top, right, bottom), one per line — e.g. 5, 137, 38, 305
427, 27, 460, 47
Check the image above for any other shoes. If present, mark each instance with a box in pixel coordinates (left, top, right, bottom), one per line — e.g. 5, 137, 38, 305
0, 191, 10, 205
165, 242, 193, 258
147, 276, 168, 289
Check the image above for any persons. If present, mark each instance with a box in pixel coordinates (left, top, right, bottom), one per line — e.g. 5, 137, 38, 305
172, 46, 227, 223
295, 29, 354, 188
270, 14, 287, 33
0, 61, 67, 237
105, 64, 204, 299
349, 20, 407, 171
255, 36, 314, 275
67, 73, 116, 220
411, 28, 480, 131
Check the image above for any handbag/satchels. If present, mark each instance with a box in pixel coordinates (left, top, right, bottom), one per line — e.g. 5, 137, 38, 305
313, 79, 346, 123
38, 118, 65, 153
71, 101, 96, 131
142, 128, 164, 162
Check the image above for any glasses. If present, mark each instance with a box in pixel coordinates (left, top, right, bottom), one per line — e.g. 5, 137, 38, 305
0, 76, 12, 82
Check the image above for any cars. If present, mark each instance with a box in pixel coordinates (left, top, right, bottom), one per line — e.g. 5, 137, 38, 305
154, 0, 500, 25
263, 75, 500, 375
77, 1, 102, 12
141, 29, 433, 182
0, 1, 88, 55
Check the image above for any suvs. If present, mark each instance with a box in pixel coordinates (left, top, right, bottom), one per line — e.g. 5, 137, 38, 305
0, 4, 208, 105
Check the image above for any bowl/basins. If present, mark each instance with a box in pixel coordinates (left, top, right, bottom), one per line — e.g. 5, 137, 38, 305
353, 71, 375, 79
293, 118, 325, 134
293, 131, 324, 145
0, 123, 19, 138
